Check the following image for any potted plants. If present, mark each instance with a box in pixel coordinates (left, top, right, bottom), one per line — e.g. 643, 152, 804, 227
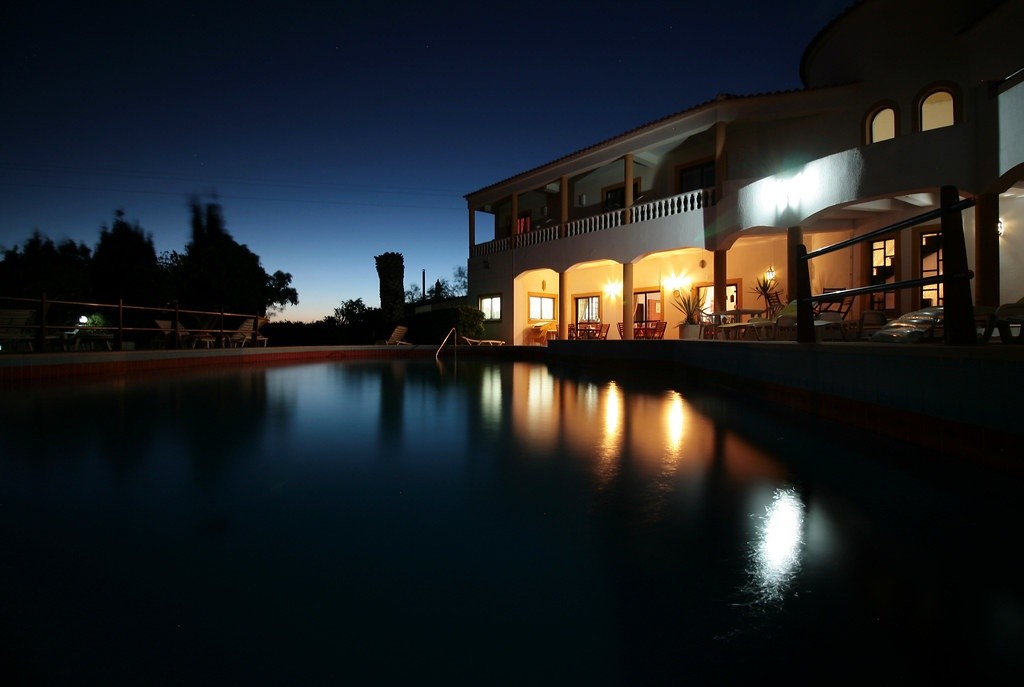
671, 288, 714, 339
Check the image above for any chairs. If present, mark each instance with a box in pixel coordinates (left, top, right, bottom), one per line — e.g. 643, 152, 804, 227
461, 335, 505, 346
222, 317, 270, 348
617, 320, 667, 340
718, 288, 886, 341
385, 325, 412, 346
0, 308, 114, 352
556, 324, 610, 340
981, 297, 1024, 344
156, 320, 216, 350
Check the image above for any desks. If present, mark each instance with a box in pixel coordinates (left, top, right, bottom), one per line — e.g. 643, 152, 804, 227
634, 320, 657, 339
547, 330, 557, 340
578, 322, 600, 339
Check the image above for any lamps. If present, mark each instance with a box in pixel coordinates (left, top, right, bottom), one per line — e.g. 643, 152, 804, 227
765, 266, 775, 280
